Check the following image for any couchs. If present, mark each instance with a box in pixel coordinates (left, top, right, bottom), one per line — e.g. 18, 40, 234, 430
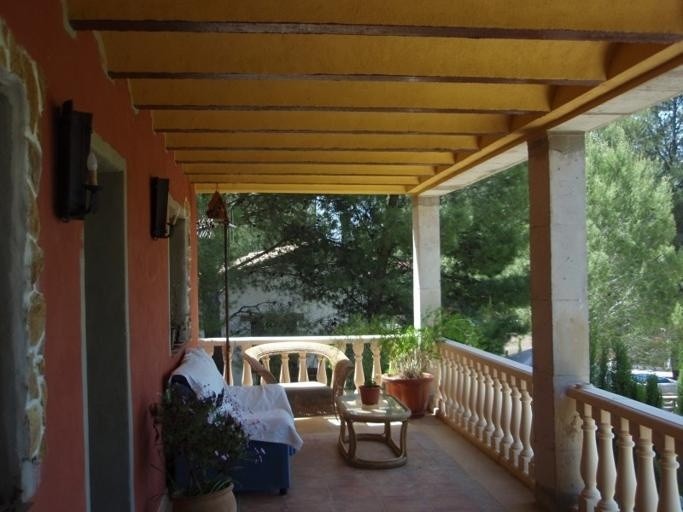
164, 343, 304, 498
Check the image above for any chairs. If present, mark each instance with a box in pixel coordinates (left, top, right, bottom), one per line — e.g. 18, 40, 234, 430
241, 339, 357, 423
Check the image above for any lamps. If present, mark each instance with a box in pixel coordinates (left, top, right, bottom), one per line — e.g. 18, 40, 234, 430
51, 96, 104, 224
149, 175, 176, 242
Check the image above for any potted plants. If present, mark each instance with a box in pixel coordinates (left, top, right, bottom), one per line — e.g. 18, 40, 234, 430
140, 381, 258, 512
358, 374, 383, 407
373, 322, 441, 421
